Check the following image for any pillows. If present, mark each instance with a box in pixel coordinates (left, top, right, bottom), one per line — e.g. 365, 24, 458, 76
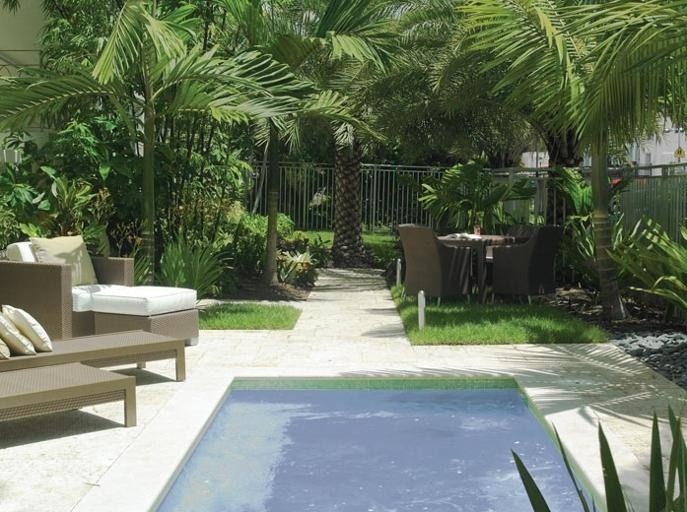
30, 234, 99, 288
0, 305, 53, 360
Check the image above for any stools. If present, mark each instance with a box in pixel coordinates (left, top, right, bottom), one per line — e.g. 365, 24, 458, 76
90, 284, 200, 347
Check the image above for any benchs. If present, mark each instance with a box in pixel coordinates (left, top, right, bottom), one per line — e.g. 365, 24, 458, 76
0, 329, 187, 427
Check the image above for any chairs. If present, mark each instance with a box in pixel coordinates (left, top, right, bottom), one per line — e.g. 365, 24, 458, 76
397, 223, 563, 309
0, 242, 134, 339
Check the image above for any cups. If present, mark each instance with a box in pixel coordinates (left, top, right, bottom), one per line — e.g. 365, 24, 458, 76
474, 225, 480, 237
